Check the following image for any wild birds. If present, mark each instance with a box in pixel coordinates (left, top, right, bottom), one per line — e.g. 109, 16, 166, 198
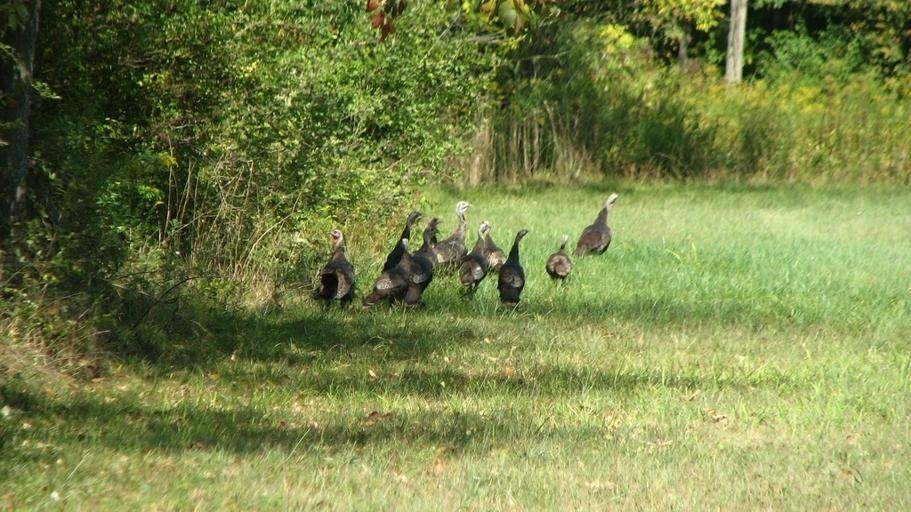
363, 201, 528, 307
308, 230, 355, 308
573, 192, 618, 256
546, 234, 572, 280
